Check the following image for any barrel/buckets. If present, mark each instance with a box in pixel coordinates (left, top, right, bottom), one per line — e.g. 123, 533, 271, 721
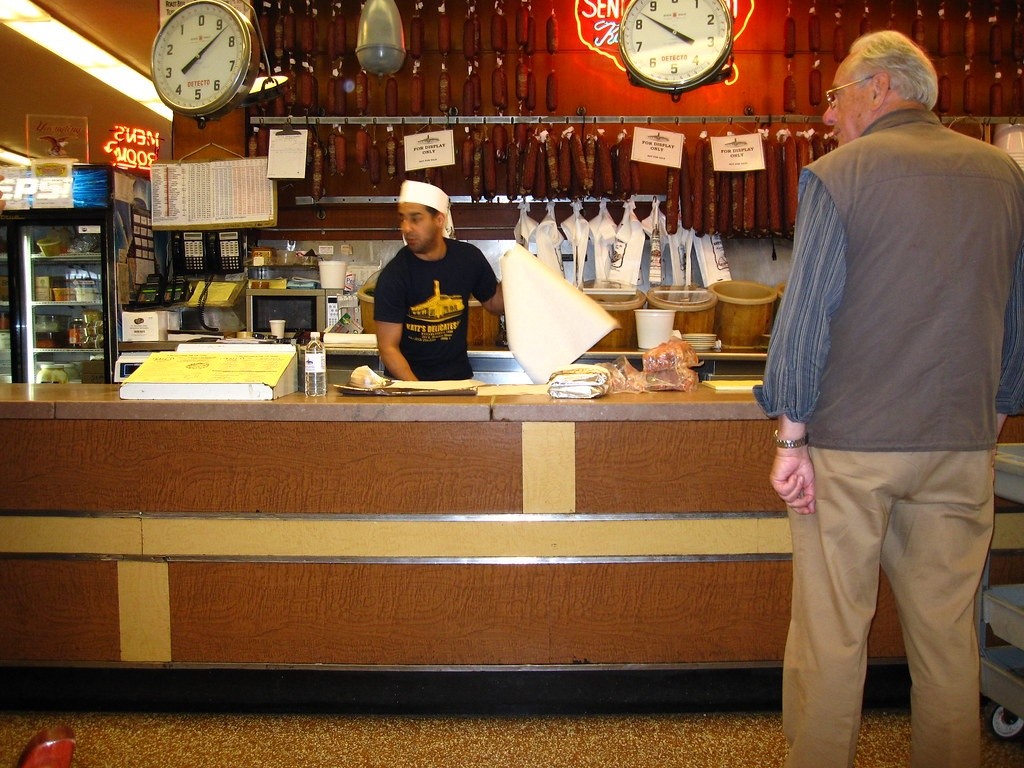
581, 280, 646, 350
707, 281, 777, 350
633, 309, 677, 349
318, 261, 347, 290
647, 285, 718, 333
466, 300, 499, 346
358, 269, 383, 334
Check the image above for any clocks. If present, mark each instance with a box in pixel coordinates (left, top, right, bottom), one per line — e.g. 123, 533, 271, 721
617, 0, 733, 94
150, 0, 260, 129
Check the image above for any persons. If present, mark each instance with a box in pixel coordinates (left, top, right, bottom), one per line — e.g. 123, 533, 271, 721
753, 30, 1024, 768
373, 181, 505, 381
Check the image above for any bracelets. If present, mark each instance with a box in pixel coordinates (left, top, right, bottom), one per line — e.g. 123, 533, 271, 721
774, 430, 809, 448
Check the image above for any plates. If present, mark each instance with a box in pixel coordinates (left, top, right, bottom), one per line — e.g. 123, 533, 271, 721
681, 334, 717, 352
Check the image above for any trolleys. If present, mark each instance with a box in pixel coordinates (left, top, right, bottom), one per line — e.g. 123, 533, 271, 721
971, 443, 1024, 740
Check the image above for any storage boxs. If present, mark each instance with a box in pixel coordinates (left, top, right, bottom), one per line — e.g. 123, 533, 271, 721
71, 163, 112, 209
30, 158, 78, 208
81, 360, 105, 384
122, 310, 179, 342
114, 171, 136, 204
36, 276, 67, 300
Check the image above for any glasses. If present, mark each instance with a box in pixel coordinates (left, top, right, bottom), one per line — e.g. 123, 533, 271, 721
825, 74, 891, 110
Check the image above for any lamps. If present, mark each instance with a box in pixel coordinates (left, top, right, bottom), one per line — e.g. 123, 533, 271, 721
0, 0, 173, 123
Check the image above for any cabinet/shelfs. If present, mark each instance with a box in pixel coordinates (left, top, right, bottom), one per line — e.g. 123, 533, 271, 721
979, 441, 1024, 721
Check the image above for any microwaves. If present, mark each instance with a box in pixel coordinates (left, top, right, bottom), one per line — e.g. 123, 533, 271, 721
245, 288, 343, 339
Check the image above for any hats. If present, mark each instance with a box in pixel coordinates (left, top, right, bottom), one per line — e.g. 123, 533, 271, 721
397, 181, 448, 215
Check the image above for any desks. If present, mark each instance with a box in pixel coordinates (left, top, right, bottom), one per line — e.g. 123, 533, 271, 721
117, 341, 769, 376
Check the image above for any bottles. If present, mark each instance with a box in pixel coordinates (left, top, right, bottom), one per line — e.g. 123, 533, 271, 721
299, 339, 309, 392
36, 314, 62, 348
305, 332, 326, 397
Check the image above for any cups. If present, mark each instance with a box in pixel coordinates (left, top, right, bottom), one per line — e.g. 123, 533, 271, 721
74, 288, 96, 301
35, 276, 50, 300
269, 320, 286, 339
36, 237, 62, 256
53, 287, 69, 301
